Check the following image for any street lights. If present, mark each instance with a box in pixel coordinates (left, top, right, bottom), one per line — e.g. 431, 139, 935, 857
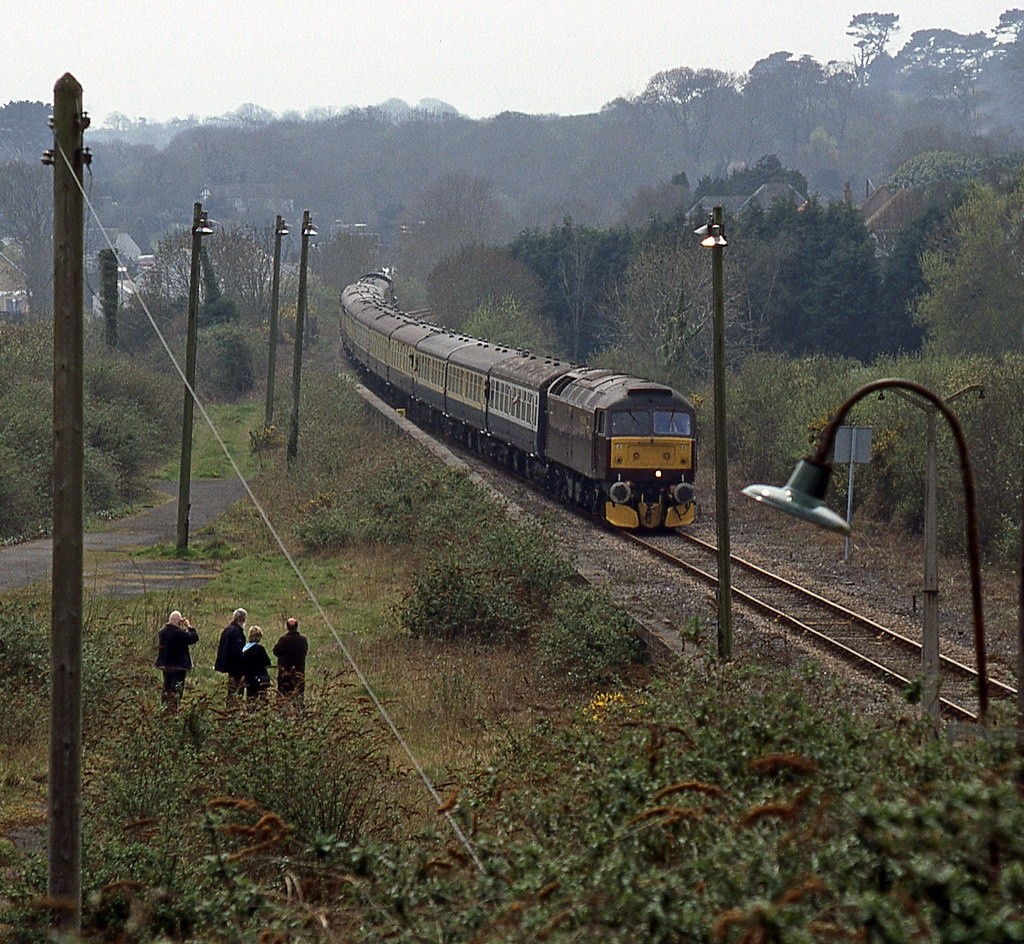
692, 206, 734, 663
264, 213, 291, 450
286, 209, 320, 471
175, 200, 224, 557
739, 377, 1003, 875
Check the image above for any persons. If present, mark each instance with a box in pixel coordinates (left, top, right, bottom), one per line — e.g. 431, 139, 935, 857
273, 619, 309, 710
154, 611, 200, 709
215, 607, 249, 700
239, 625, 273, 705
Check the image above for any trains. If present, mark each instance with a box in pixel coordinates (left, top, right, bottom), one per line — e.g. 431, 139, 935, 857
337, 268, 698, 527
